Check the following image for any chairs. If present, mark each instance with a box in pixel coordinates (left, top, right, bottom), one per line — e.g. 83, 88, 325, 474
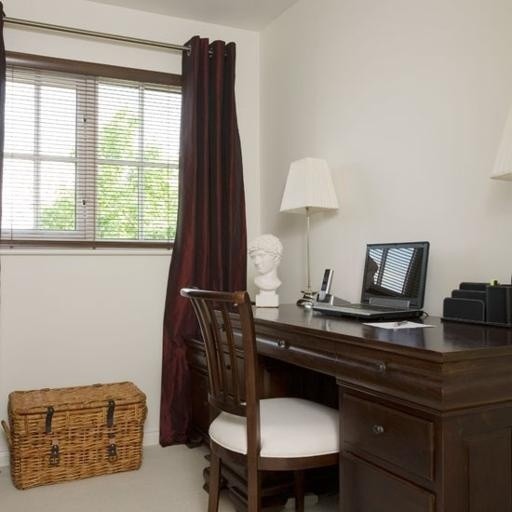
179, 285, 340, 512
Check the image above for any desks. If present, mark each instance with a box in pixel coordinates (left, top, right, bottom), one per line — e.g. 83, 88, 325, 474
182, 297, 512, 512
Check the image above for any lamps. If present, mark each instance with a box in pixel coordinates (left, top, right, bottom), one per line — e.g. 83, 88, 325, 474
279, 157, 338, 306
489, 108, 512, 182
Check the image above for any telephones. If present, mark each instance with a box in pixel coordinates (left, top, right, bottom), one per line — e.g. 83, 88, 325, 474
314, 269, 333, 306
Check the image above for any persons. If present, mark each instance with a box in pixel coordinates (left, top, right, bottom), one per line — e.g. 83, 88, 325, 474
248, 234, 284, 290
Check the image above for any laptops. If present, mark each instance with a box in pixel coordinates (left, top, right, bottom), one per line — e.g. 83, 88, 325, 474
312, 241, 430, 320
